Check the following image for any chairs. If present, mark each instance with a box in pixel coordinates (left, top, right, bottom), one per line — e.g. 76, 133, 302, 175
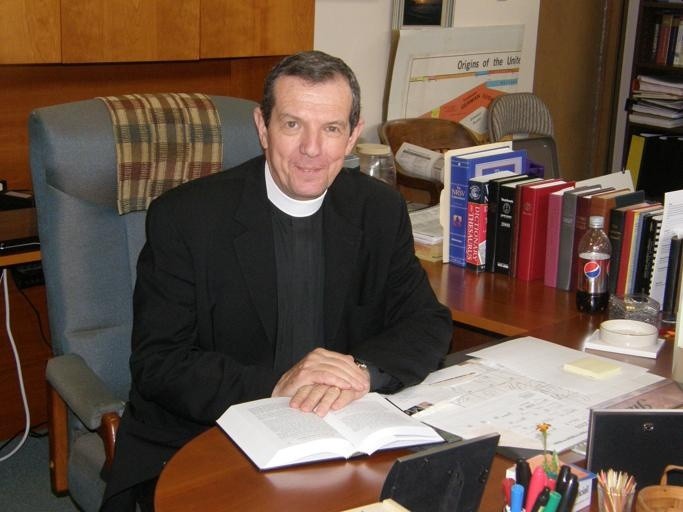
30, 92, 264, 510
377, 92, 559, 207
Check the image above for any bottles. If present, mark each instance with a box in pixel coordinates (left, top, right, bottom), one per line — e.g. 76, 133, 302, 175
354, 141, 398, 187
574, 214, 612, 314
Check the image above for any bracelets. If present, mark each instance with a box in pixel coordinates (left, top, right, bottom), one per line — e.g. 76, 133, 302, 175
354, 359, 368, 369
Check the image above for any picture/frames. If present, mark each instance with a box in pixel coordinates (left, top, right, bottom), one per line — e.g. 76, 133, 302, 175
392, 1, 454, 41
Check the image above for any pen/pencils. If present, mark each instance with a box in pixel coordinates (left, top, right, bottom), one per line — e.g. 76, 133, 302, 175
597, 468, 637, 512
502, 457, 578, 512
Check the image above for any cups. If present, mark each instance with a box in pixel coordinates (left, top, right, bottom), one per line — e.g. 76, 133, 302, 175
596, 481, 636, 512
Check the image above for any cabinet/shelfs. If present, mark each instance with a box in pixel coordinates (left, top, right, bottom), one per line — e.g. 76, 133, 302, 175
611, 0, 683, 176
0, 0, 315, 64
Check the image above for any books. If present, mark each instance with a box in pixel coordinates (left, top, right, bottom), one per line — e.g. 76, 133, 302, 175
616, 0, 682, 133
214, 390, 447, 472
408, 141, 683, 317
565, 356, 622, 378
585, 329, 667, 360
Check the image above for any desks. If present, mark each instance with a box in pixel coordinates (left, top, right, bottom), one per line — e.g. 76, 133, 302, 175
153, 298, 682, 511
404, 199, 585, 337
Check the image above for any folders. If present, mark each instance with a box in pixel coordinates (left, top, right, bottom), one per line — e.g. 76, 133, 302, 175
623, 130, 683, 206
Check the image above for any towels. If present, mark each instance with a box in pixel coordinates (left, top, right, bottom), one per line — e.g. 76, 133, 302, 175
94, 92, 223, 216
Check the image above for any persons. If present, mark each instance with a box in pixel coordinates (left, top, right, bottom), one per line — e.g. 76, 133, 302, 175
98, 48, 457, 512
